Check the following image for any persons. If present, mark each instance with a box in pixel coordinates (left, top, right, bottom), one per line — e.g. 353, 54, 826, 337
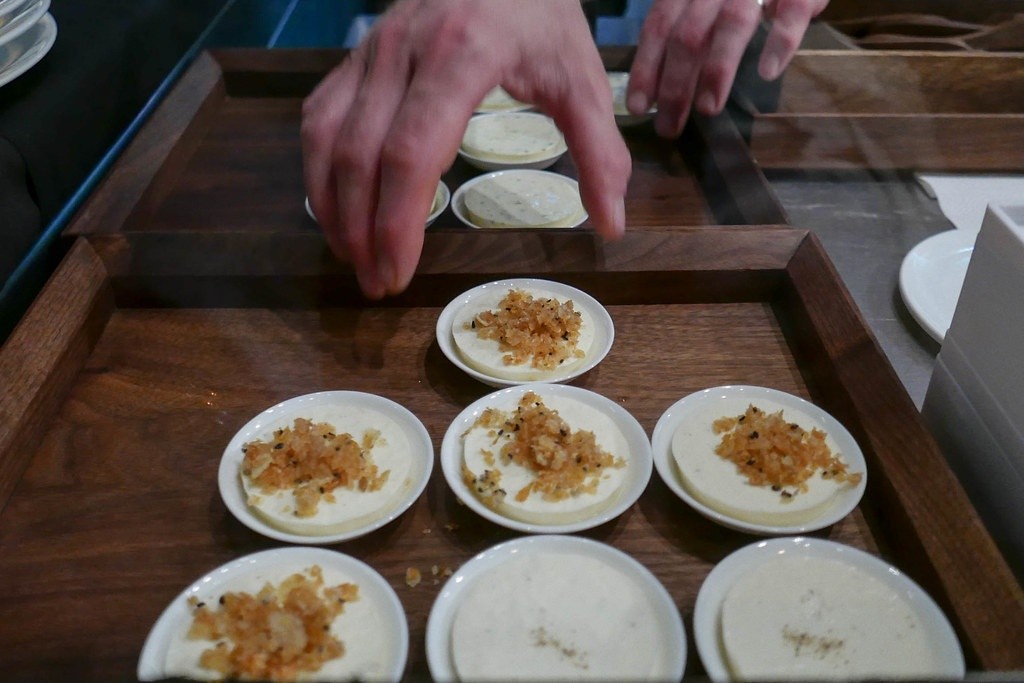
302, 0, 836, 297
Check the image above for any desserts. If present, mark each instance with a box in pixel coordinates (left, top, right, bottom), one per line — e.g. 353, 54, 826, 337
163, 287, 933, 683
432, 81, 659, 228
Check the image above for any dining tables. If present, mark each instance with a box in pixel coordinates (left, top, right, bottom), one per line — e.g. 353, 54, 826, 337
0, 0, 962, 421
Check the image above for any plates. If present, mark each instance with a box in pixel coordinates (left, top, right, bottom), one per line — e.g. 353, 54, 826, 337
419, 528, 691, 682
898, 224, 988, 343
431, 276, 615, 387
209, 384, 431, 546
0, 10, 56, 91
442, 376, 655, 533
450, 168, 589, 228
303, 179, 450, 231
136, 539, 411, 683
652, 381, 865, 535
459, 112, 567, 168
696, 535, 965, 683
606, 72, 660, 125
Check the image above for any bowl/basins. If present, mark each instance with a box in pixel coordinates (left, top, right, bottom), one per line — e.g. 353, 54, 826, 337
1, 1, 50, 74
